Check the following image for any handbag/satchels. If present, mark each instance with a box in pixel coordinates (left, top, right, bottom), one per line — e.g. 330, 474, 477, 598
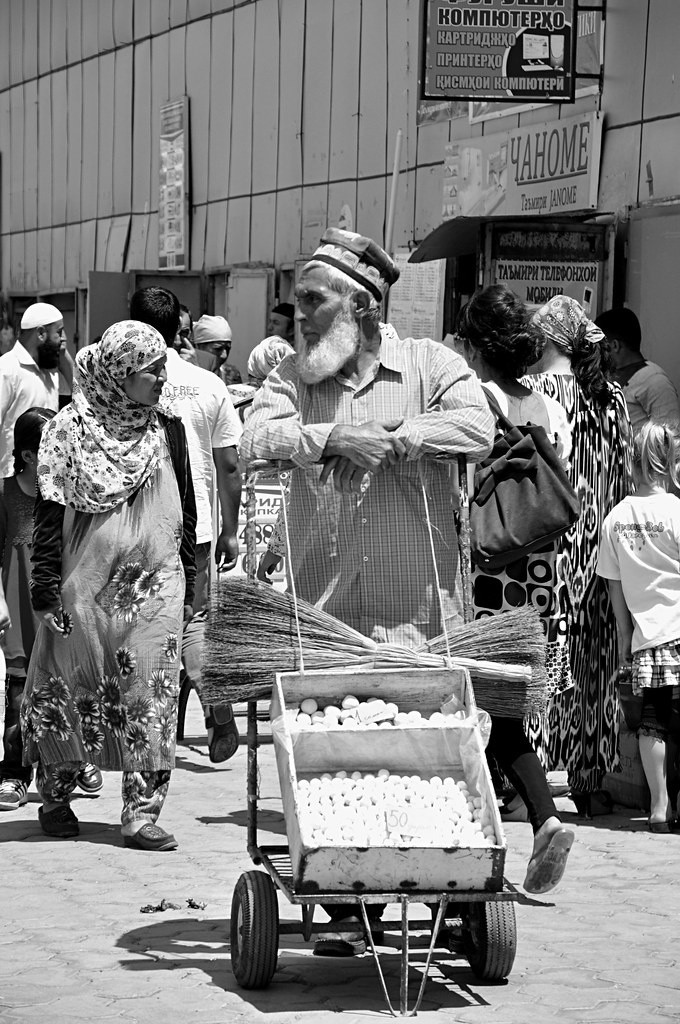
467, 384, 580, 568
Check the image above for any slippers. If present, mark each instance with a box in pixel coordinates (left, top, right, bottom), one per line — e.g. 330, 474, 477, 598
648, 811, 678, 833
524, 827, 575, 893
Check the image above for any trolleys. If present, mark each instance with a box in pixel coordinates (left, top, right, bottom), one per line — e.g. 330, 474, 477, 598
230, 451, 520, 1019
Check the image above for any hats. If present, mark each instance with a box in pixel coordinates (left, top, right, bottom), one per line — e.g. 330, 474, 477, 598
312, 226, 401, 303
271, 302, 295, 320
19, 302, 64, 330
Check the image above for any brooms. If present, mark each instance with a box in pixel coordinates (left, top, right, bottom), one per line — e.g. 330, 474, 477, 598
412, 602, 548, 717
201, 576, 532, 707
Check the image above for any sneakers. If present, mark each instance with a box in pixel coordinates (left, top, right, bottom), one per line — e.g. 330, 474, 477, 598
0, 778, 28, 810
75, 760, 104, 791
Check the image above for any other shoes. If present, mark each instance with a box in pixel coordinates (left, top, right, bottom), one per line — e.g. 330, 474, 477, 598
432, 917, 466, 954
124, 821, 179, 851
204, 695, 240, 763
498, 790, 531, 822
313, 912, 383, 956
571, 791, 612, 817
38, 804, 79, 838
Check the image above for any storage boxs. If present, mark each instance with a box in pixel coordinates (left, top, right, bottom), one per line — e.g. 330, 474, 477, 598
270, 667, 506, 893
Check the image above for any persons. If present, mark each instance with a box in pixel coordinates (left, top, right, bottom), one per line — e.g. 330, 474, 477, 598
0, 285, 299, 807
500, 295, 680, 832
21, 319, 197, 851
459, 286, 577, 894
236, 226, 494, 956
